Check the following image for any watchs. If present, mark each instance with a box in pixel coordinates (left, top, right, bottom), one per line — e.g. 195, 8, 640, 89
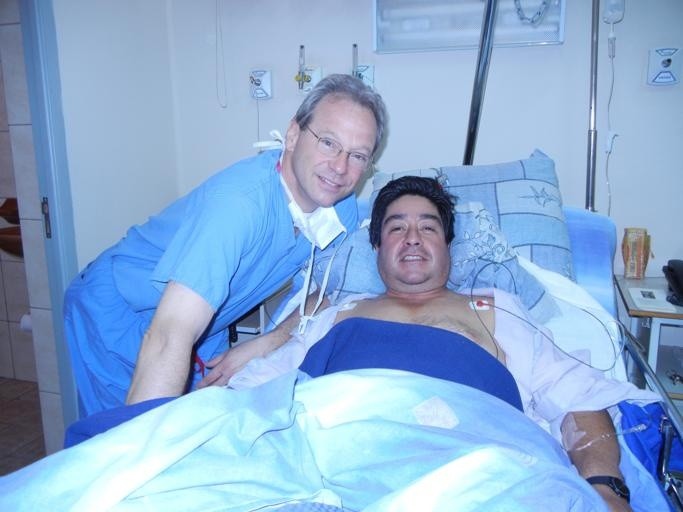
585, 475, 629, 505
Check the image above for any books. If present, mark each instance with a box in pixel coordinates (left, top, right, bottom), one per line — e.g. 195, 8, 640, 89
626, 285, 678, 315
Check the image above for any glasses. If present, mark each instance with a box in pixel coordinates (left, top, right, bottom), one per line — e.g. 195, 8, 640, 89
303, 124, 374, 173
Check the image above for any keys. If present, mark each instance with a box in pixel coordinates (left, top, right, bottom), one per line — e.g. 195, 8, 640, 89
670, 373, 678, 385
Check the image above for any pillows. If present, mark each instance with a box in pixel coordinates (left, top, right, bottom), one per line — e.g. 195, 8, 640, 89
333, 148, 574, 289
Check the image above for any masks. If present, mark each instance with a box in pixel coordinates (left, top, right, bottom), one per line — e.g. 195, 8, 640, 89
287, 201, 344, 250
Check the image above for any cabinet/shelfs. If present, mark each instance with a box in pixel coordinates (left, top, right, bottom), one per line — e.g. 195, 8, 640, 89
615, 275, 683, 404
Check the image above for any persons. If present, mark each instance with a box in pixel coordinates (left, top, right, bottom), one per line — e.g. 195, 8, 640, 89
222, 173, 633, 512
63, 71, 386, 420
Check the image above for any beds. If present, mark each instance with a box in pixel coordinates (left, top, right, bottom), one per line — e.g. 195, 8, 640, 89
0, 198, 683, 510
